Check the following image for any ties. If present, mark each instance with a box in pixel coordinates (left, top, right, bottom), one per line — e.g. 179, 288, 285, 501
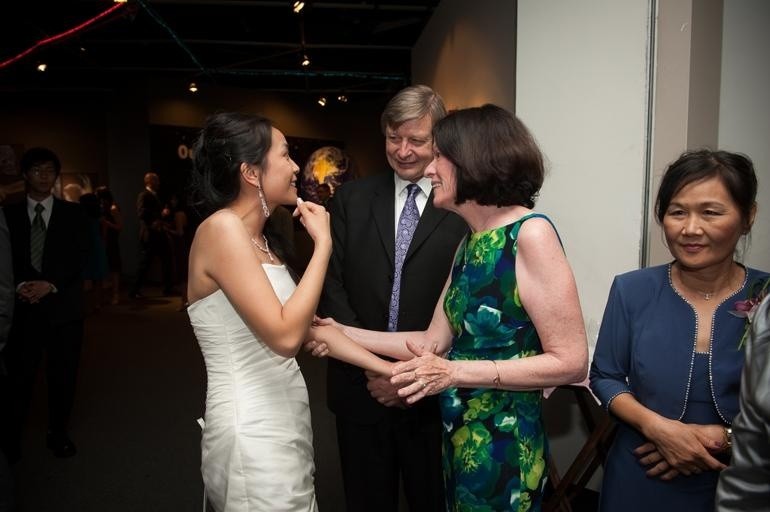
388, 184, 423, 333
30, 203, 47, 273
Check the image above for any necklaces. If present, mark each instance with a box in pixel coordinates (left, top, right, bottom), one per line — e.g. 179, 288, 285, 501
672, 264, 741, 300
249, 233, 275, 261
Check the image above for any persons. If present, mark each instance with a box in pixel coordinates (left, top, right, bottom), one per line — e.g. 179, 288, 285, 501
1, 145, 89, 354
297, 83, 470, 512
296, 102, 589, 511
185, 112, 334, 512
135, 172, 175, 258
96, 185, 124, 305
589, 148, 770, 512
714, 293, 770, 512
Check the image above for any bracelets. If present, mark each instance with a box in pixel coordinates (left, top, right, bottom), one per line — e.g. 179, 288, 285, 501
722, 427, 732, 451
490, 360, 501, 391
